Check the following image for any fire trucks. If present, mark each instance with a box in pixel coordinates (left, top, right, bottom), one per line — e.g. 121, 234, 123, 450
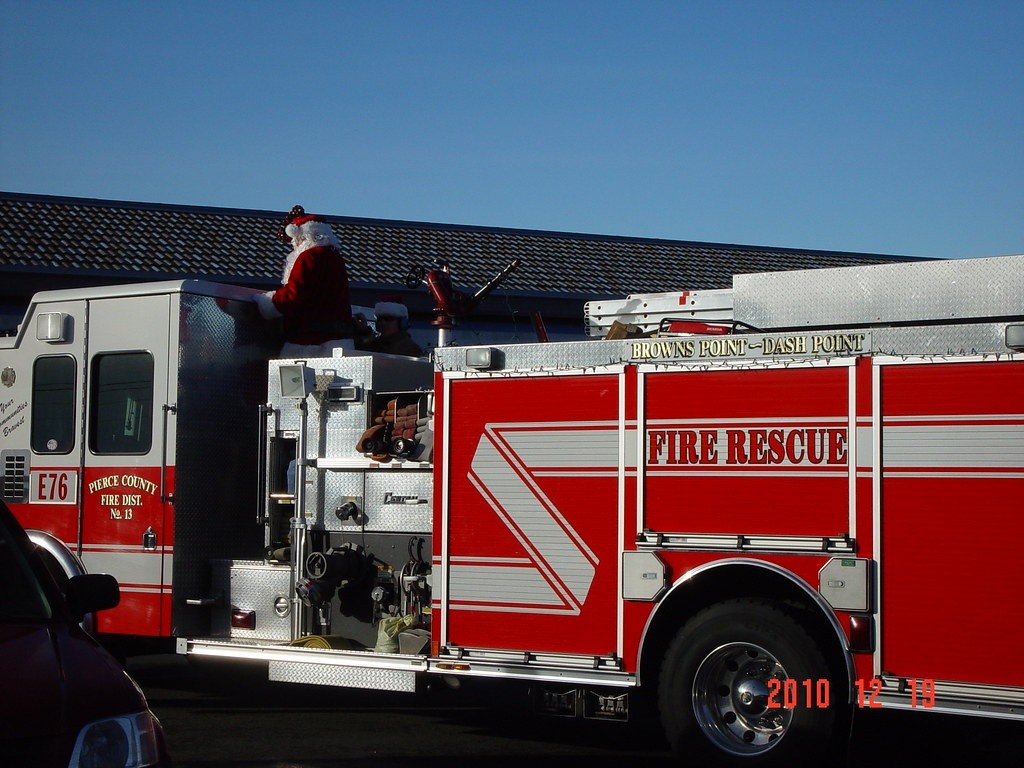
1, 254, 1024, 768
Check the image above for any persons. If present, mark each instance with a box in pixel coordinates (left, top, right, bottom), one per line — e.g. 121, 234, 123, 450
264, 215, 355, 358
370, 295, 422, 357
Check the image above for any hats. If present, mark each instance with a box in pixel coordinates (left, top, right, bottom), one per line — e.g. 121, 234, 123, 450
375, 295, 408, 320
285, 214, 333, 239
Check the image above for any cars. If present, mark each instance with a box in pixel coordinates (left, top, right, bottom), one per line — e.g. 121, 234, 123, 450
1, 500, 169, 767
